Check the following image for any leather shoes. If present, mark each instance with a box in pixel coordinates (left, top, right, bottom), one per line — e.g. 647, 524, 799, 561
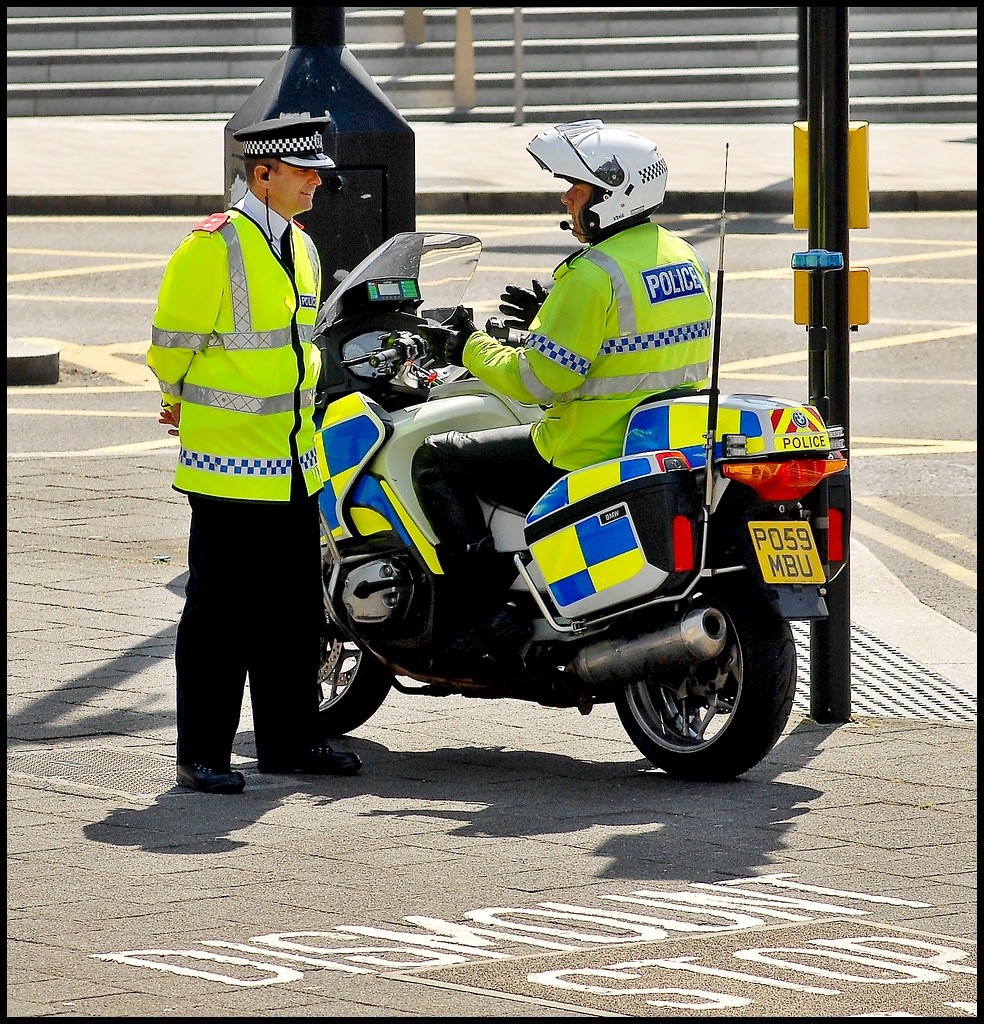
177, 762, 243, 796
264, 744, 363, 776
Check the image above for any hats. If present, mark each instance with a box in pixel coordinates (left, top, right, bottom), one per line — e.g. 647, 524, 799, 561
232, 117, 336, 169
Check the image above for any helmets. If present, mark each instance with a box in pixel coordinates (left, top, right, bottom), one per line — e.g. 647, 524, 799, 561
526, 118, 668, 241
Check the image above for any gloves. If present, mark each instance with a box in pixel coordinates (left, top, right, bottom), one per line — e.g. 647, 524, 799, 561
500, 280, 550, 332
444, 306, 478, 367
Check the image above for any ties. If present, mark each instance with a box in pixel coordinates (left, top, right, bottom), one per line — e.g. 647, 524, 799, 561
281, 224, 295, 278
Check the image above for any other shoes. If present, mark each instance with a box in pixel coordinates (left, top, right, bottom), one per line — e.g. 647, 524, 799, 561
430, 605, 532, 674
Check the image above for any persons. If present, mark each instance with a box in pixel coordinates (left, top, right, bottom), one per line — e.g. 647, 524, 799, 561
411, 119, 712, 673
143, 116, 363, 795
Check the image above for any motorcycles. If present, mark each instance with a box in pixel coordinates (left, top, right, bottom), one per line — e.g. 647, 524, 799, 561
312, 232, 848, 781
